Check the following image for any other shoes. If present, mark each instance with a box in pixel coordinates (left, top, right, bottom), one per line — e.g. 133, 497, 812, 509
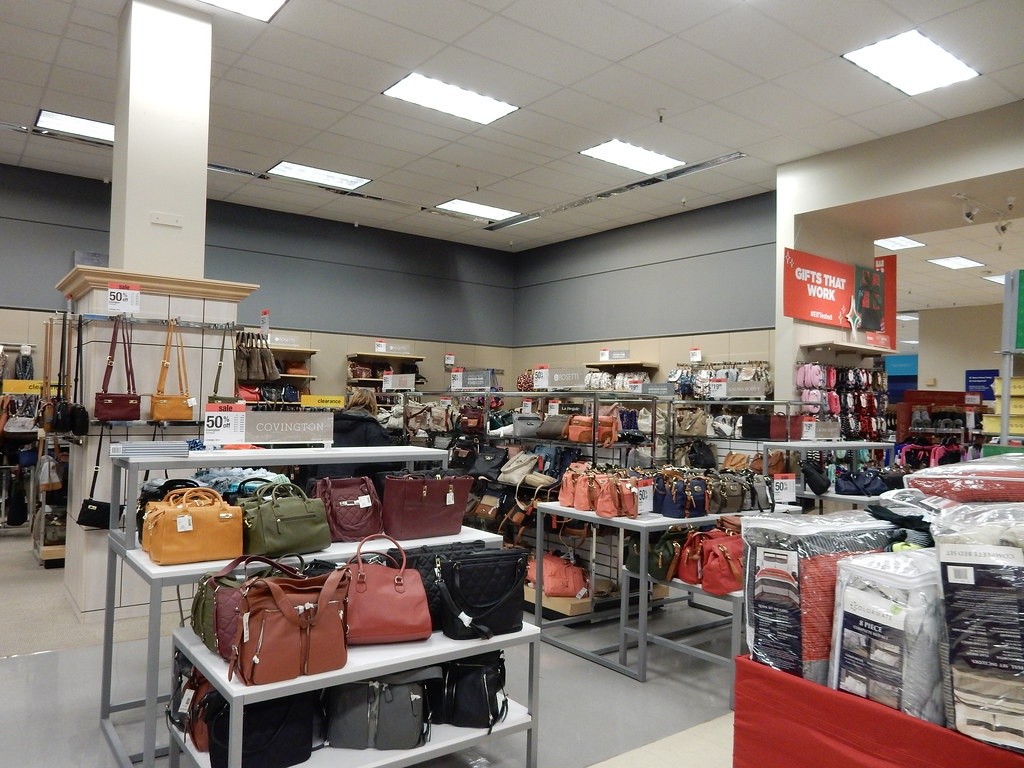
911, 411, 984, 431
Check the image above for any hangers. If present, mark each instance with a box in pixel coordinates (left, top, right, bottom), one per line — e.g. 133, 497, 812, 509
900, 426, 980, 449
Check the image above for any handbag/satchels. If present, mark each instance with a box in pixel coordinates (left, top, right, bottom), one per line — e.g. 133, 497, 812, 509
796, 460, 831, 496
0, 311, 817, 768
835, 470, 888, 497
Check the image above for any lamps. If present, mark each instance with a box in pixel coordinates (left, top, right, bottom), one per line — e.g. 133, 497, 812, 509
952, 193, 1017, 237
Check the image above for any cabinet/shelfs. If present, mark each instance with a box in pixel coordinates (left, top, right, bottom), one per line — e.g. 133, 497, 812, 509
239, 346, 320, 405
346, 340, 901, 681
981, 268, 1024, 456
100, 446, 537, 768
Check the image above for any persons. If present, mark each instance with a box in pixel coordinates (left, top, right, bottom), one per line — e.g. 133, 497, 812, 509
318, 389, 392, 475
